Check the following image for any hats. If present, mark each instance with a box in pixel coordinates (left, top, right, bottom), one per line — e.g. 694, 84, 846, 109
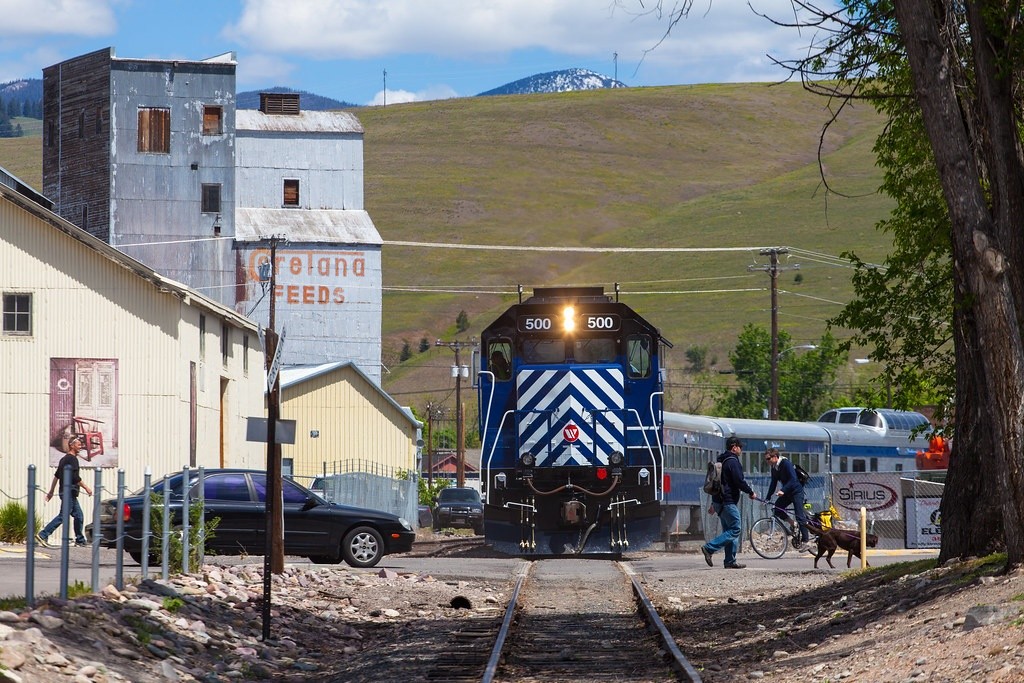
729, 438, 744, 446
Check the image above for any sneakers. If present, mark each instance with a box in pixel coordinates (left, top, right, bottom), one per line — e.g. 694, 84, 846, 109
792, 519, 797, 532
36, 533, 52, 548
799, 542, 811, 552
75, 542, 93, 549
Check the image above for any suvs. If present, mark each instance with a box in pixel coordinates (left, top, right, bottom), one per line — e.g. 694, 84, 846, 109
431, 485, 486, 531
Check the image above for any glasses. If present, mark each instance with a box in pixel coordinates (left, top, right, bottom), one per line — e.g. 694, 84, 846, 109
70, 436, 80, 443
765, 456, 773, 461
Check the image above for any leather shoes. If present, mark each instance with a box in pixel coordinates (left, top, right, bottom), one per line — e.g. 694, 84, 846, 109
702, 545, 713, 566
725, 560, 746, 568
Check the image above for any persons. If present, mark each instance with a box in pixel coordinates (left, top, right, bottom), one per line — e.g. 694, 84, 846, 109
700, 437, 756, 569
762, 447, 813, 553
36, 438, 92, 547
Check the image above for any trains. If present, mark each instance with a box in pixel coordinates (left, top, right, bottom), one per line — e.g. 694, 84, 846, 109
470, 283, 952, 561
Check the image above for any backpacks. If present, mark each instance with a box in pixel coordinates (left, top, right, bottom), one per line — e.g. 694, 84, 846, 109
793, 463, 810, 488
703, 455, 739, 495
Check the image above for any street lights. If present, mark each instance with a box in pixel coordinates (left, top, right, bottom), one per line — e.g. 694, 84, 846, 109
770, 344, 819, 419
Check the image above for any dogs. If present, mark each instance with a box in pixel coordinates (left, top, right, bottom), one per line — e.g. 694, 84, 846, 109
802, 521, 878, 568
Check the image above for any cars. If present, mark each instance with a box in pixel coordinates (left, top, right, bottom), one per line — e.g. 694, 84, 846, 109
84, 467, 415, 568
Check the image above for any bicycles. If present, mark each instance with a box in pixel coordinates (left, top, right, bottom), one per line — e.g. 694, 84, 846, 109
750, 499, 835, 559
